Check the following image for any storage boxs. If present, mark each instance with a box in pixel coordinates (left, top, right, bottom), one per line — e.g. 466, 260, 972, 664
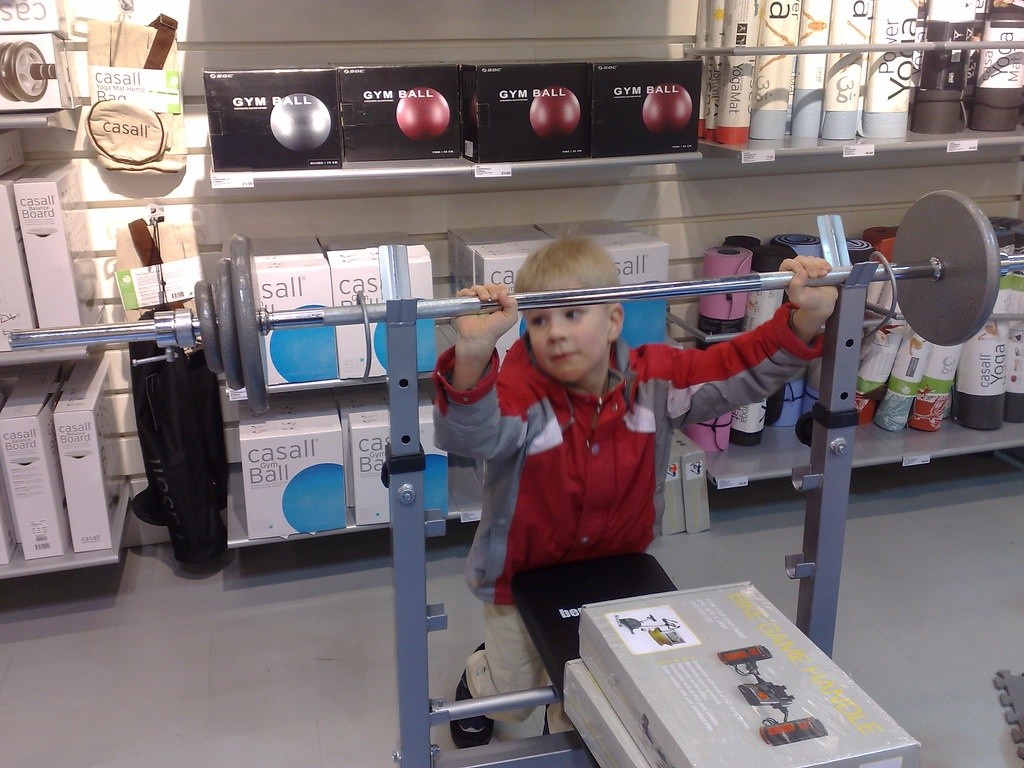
461, 60, 593, 164
562, 579, 922, 767
218, 221, 713, 543
591, 57, 703, 159
329, 62, 464, 162
200, 65, 343, 169
0, 1, 128, 566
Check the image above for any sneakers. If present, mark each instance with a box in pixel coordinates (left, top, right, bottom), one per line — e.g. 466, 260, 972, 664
450, 643, 494, 748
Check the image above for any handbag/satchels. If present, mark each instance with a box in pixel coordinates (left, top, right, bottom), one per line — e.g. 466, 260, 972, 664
87, 14, 187, 174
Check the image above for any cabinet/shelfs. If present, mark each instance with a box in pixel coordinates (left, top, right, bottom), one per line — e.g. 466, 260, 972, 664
208, 153, 705, 550
0, 108, 131, 580
667, 41, 1024, 488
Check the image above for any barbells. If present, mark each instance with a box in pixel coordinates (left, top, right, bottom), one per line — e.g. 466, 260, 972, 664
6, 189, 1024, 417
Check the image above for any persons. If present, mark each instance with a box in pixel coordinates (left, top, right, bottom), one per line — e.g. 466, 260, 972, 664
436, 233, 838, 749
